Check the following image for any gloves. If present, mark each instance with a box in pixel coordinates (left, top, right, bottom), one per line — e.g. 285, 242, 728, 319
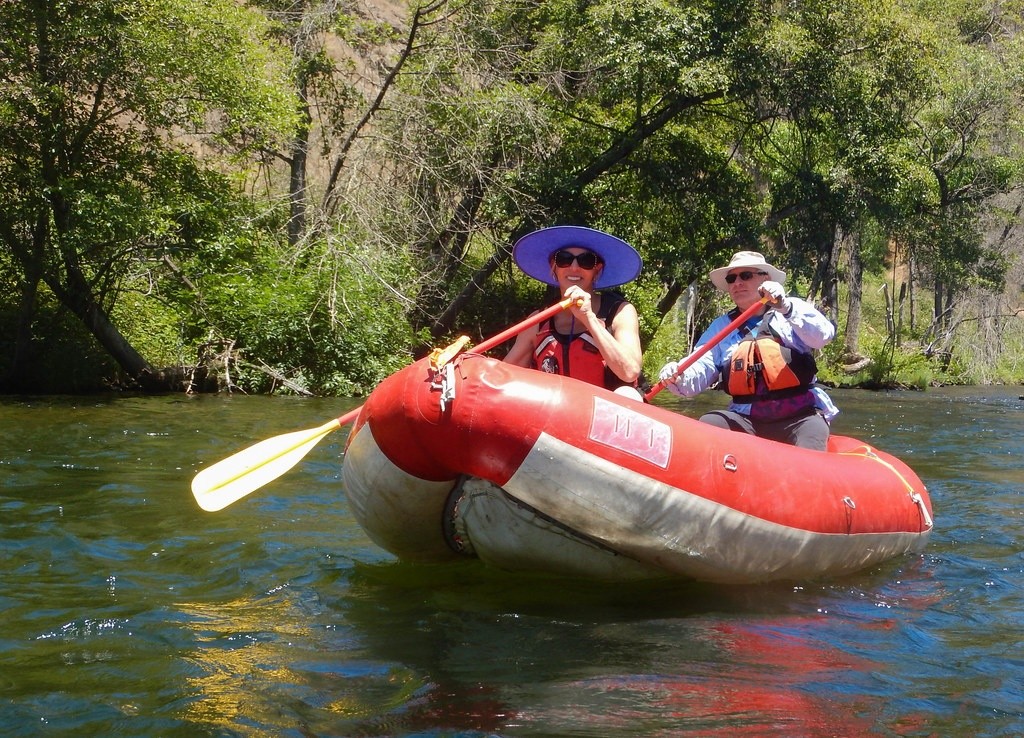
657, 361, 684, 388
757, 280, 792, 315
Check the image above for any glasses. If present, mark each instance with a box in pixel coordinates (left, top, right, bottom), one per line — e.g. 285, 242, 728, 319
551, 250, 602, 270
725, 272, 769, 283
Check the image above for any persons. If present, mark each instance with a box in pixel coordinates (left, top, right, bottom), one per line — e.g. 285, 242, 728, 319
501, 226, 644, 403
658, 250, 839, 451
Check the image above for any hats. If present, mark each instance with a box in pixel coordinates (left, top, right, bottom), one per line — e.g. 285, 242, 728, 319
708, 251, 786, 292
512, 225, 643, 290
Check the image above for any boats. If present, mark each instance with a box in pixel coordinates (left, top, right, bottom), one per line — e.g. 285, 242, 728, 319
342, 334, 938, 600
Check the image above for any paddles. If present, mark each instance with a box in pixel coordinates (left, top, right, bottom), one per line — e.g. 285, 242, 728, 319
641, 289, 772, 405
183, 297, 574, 513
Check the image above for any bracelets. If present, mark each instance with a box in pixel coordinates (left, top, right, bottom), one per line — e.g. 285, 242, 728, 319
784, 302, 793, 316
